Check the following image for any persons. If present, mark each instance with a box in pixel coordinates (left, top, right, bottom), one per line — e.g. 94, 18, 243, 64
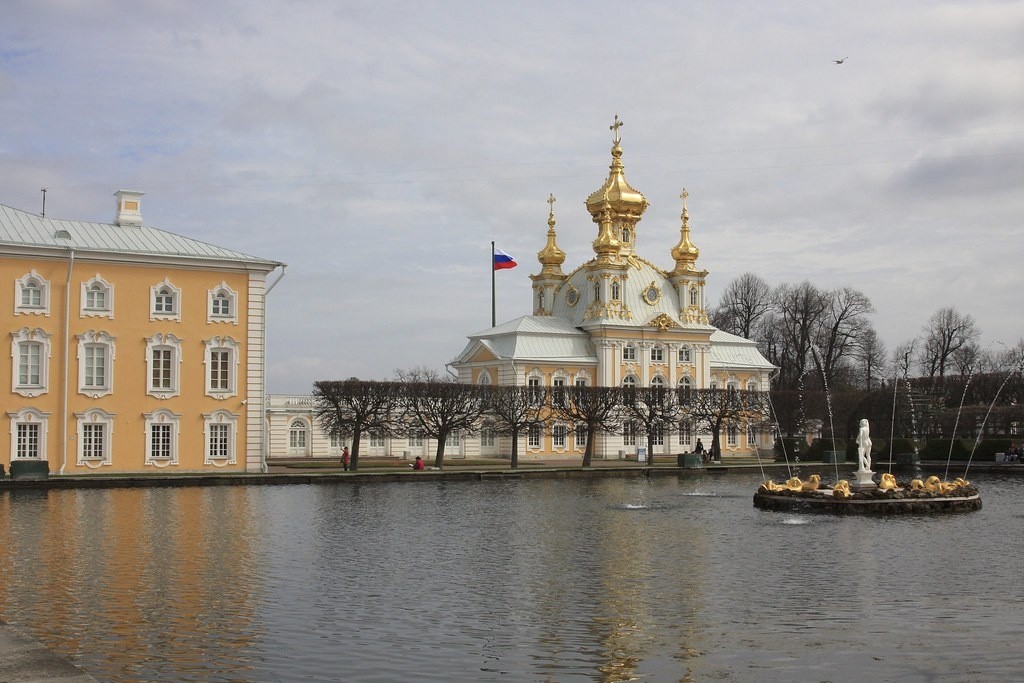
857, 419, 871, 471
1005, 442, 1024, 462
684, 438, 716, 463
412, 456, 424, 470
340, 446, 349, 471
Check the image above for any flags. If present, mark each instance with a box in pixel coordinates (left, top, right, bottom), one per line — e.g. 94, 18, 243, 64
493, 247, 518, 271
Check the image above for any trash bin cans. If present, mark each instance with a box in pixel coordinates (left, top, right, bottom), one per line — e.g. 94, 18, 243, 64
826, 451, 848, 463
10, 460, 50, 478
679, 452, 702, 467
857, 452, 878, 464
898, 452, 917, 463
